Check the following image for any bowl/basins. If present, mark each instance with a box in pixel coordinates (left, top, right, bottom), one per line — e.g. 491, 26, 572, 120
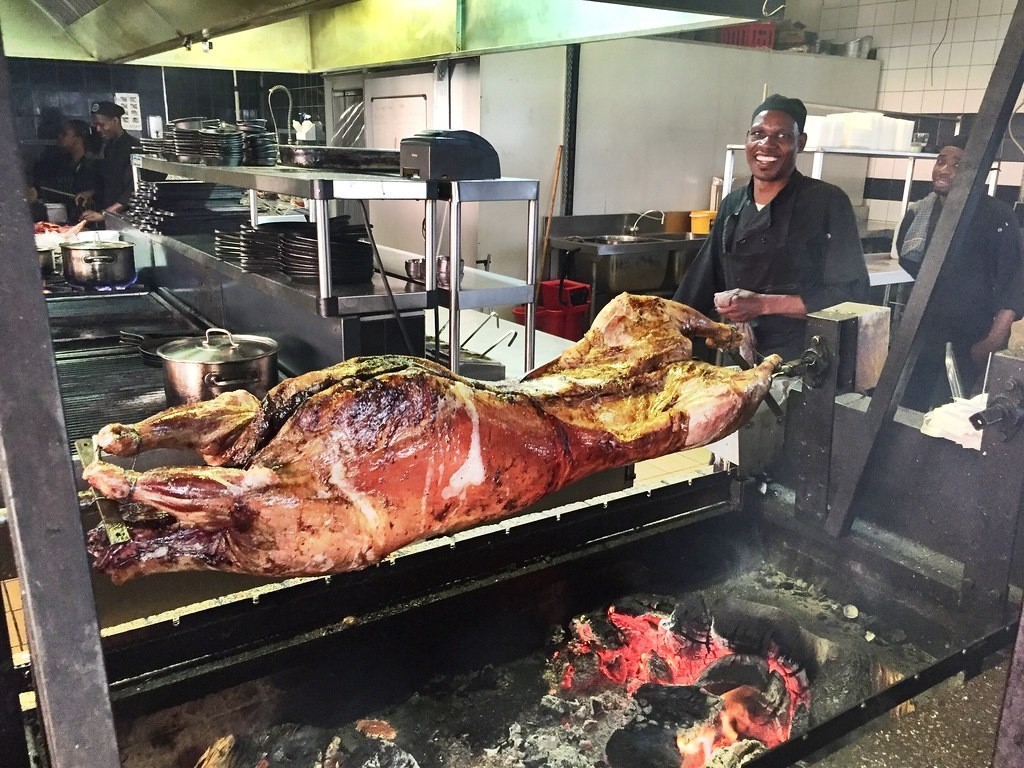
138, 113, 279, 167
405, 255, 464, 287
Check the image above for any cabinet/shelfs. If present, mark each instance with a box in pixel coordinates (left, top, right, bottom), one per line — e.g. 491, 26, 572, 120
129, 152, 542, 385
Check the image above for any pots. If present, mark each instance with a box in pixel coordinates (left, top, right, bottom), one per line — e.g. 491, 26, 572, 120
118, 330, 193, 367
156, 328, 281, 407
36, 246, 56, 273
59, 240, 136, 287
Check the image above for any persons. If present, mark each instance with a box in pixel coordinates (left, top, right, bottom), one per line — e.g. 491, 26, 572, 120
672, 93, 870, 368
896, 133, 1024, 410
33, 98, 142, 230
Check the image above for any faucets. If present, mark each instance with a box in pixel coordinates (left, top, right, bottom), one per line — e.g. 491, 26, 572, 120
628, 210, 665, 236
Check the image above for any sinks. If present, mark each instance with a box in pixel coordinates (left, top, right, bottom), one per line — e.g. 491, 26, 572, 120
640, 232, 710, 290
564, 234, 674, 294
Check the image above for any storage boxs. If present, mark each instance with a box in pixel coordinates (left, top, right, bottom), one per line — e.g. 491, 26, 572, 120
776, 30, 818, 54
721, 22, 775, 49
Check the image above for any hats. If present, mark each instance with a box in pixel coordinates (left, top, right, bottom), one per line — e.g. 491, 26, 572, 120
91, 102, 125, 118
945, 136, 968, 149
752, 94, 807, 134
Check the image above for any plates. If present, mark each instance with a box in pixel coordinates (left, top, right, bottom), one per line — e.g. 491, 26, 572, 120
212, 214, 376, 284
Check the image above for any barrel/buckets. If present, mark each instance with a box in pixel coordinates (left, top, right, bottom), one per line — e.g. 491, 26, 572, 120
689, 211, 718, 234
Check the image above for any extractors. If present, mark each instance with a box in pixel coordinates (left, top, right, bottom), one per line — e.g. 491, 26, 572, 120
0, 0, 362, 65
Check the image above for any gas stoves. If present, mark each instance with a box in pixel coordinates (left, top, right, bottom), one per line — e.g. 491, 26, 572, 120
40, 258, 150, 301
